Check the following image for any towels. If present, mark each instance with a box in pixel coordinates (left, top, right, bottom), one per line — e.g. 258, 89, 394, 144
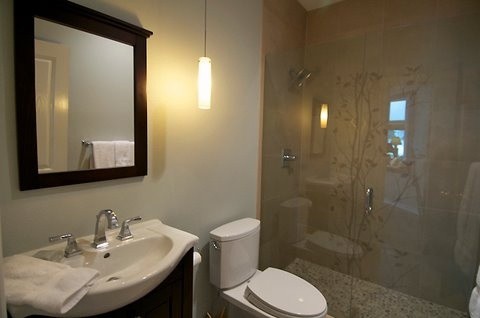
0, 254, 99, 313
89, 139, 134, 168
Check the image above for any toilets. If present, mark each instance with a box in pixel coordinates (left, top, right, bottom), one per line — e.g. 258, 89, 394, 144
209, 216, 329, 318
278, 196, 364, 276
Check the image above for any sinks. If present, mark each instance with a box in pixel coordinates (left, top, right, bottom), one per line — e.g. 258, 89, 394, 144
51, 233, 174, 285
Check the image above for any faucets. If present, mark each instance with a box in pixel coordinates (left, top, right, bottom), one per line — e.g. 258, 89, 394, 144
92, 207, 119, 250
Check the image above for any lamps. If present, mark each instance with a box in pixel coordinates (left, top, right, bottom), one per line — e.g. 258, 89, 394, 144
319, 105, 328, 130
198, 0, 212, 108
